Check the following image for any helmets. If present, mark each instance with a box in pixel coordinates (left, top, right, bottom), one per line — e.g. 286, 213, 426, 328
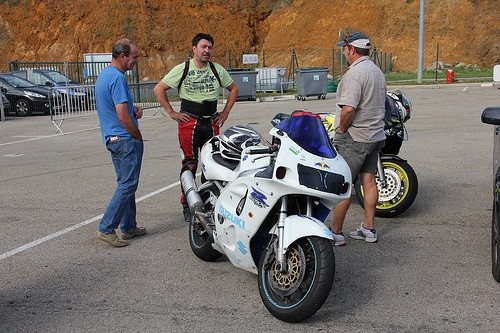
388, 90, 413, 124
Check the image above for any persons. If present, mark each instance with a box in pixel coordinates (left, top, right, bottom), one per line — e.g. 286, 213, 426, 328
327, 32, 386, 246
154, 33, 238, 222
94, 38, 147, 246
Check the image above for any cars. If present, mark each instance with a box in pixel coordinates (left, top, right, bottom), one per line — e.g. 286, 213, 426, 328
0, 67, 95, 119
481, 107, 500, 282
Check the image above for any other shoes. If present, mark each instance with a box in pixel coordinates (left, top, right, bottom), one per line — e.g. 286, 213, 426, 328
183, 208, 191, 222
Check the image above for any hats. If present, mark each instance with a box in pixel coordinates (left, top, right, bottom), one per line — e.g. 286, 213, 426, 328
335, 32, 372, 50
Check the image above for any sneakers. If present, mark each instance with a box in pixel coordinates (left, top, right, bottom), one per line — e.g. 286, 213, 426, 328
349, 222, 378, 243
328, 226, 346, 246
121, 226, 146, 240
96, 229, 130, 247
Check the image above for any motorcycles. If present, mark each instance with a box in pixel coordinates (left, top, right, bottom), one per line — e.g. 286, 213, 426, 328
180, 110, 351, 323
318, 112, 419, 218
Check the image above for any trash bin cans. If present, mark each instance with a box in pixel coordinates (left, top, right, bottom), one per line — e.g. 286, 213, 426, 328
295, 67, 329, 101
223, 67, 258, 102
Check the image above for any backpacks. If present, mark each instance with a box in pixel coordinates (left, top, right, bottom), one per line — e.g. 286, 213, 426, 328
379, 94, 409, 155
